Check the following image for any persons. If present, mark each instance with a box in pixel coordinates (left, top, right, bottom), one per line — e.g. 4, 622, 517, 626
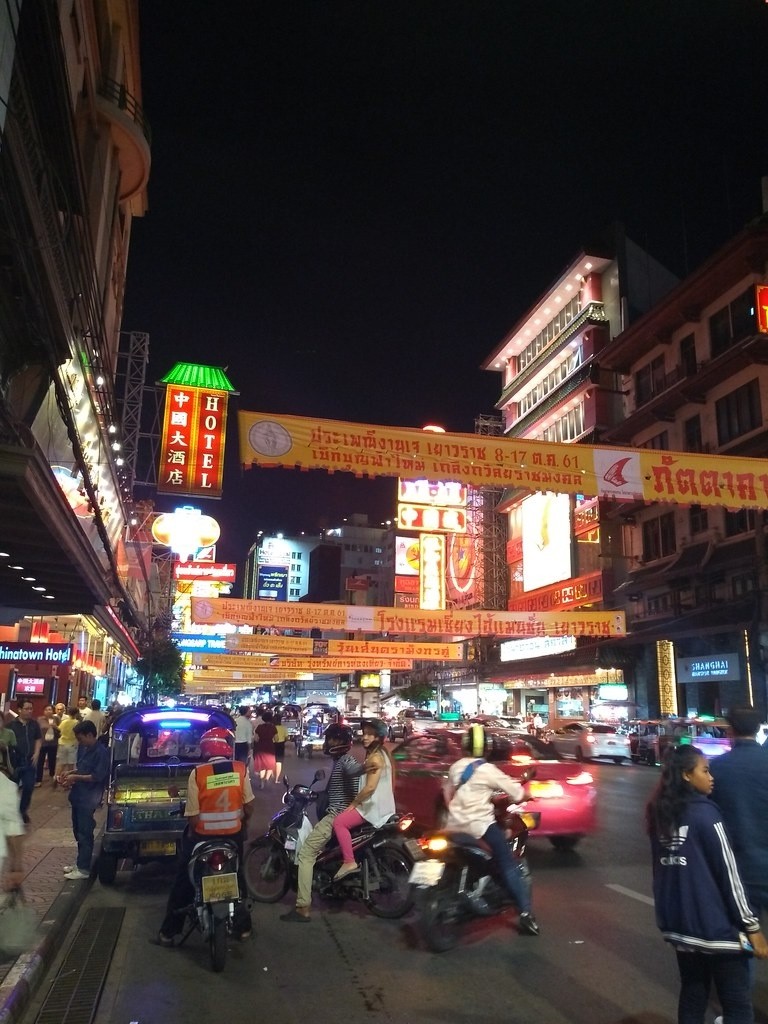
645, 745, 768, 1024
442, 723, 540, 935
333, 717, 396, 881
0, 769, 28, 892
148, 726, 254, 947
711, 699, 768, 1024
33, 704, 61, 788
77, 695, 93, 722
534, 713, 542, 728
102, 700, 299, 790
281, 723, 379, 921
83, 699, 107, 739
54, 703, 70, 728
516, 711, 524, 722
0, 711, 18, 778
59, 719, 110, 879
434, 704, 485, 720
527, 697, 535, 717
7, 700, 42, 823
618, 722, 712, 767
52, 707, 80, 790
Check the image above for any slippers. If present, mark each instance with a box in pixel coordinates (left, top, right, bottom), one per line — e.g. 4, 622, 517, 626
333, 864, 361, 882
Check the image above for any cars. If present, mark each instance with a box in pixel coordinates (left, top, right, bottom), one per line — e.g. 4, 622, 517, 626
205, 698, 525, 757
385, 727, 598, 851
623, 717, 735, 769
547, 722, 632, 765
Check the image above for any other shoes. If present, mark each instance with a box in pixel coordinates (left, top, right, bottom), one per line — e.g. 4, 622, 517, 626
159, 932, 175, 948
22, 814, 30, 826
34, 782, 41, 787
519, 914, 542, 936
49, 777, 56, 784
241, 930, 252, 938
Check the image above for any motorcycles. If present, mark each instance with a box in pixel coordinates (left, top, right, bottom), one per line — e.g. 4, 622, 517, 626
409, 764, 539, 953
241, 768, 429, 920
96, 704, 247, 884
166, 784, 254, 974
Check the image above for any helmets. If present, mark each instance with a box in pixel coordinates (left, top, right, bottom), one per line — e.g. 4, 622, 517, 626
361, 717, 388, 745
461, 726, 493, 757
200, 726, 236, 759
323, 723, 353, 755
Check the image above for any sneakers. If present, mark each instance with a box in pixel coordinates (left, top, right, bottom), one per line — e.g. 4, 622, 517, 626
64, 870, 89, 879
64, 863, 77, 872
281, 906, 310, 922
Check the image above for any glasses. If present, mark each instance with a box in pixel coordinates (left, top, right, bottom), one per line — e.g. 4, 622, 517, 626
21, 708, 33, 710
